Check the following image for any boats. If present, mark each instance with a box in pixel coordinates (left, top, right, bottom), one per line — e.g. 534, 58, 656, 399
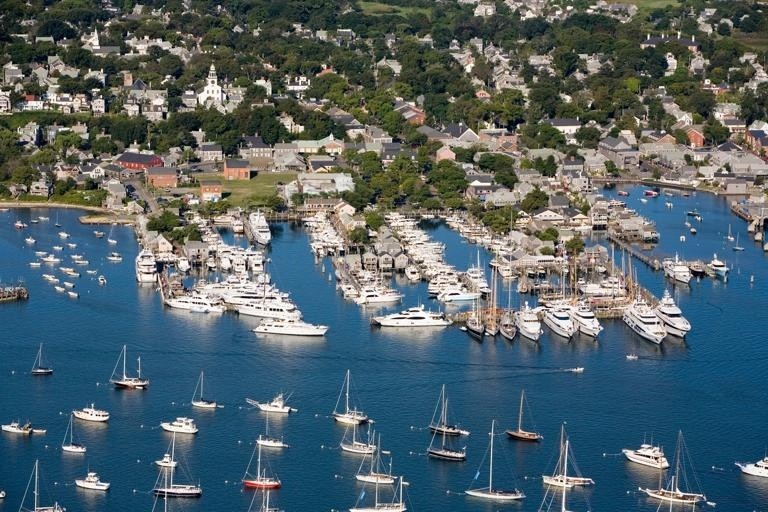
732, 452, 767, 479
617, 190, 630, 197
638, 184, 674, 210
14, 211, 125, 301
622, 286, 667, 345
678, 207, 704, 244
652, 288, 692, 338
134, 243, 158, 283
706, 252, 731, 283
163, 208, 639, 337
662, 250, 692, 284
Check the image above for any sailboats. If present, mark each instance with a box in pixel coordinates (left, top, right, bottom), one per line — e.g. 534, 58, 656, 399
1, 340, 718, 512
727, 224, 745, 252
